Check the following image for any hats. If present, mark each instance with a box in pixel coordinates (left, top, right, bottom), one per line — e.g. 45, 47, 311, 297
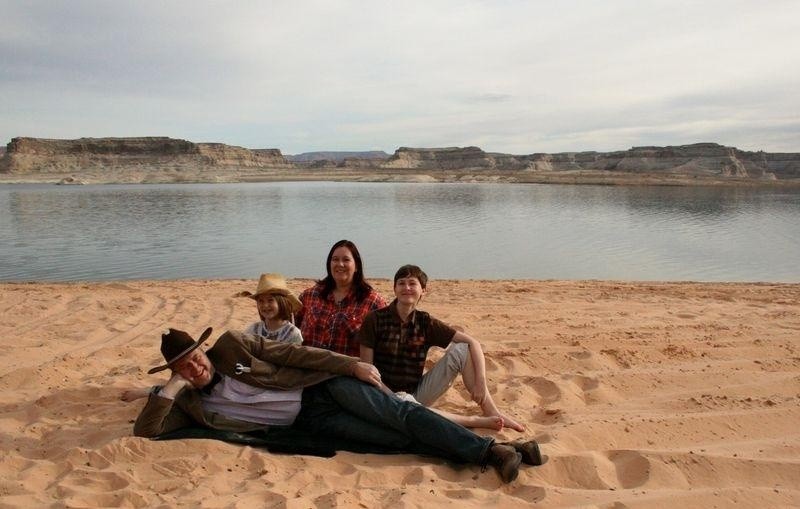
250, 272, 303, 312
148, 327, 212, 374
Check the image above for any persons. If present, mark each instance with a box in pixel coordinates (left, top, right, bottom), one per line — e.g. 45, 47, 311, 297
294, 239, 389, 362
242, 274, 303, 346
361, 265, 526, 432
134, 328, 541, 482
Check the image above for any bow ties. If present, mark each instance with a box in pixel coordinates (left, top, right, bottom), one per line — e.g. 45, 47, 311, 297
203, 372, 222, 395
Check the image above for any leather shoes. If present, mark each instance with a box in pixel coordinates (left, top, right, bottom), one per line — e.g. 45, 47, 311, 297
487, 437, 542, 484
